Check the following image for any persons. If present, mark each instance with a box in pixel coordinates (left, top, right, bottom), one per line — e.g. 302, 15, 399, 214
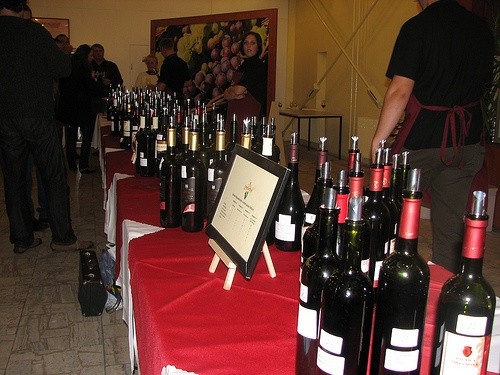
134, 55, 161, 93
22, 5, 49, 232
372, 0, 500, 271
224, 31, 267, 143
155, 39, 192, 110
92, 44, 123, 113
0, 0, 94, 253
55, 34, 97, 175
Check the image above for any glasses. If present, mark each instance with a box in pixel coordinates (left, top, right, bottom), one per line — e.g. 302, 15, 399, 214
243, 40, 258, 45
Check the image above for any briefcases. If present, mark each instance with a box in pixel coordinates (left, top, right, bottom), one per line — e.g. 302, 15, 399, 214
78, 249, 108, 317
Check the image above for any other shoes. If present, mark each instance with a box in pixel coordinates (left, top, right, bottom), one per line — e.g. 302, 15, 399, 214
68, 160, 78, 171
51, 240, 93, 251
80, 169, 96, 174
13, 237, 42, 254
33, 216, 49, 230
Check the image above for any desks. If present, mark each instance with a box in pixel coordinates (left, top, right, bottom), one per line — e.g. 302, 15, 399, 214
279, 110, 343, 160
97, 108, 500, 375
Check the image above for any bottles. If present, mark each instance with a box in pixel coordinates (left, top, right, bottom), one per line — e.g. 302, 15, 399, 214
296, 185, 341, 374
106, 84, 279, 247
302, 135, 410, 305
430, 190, 496, 374
315, 195, 374, 375
370, 169, 430, 375
274, 132, 305, 252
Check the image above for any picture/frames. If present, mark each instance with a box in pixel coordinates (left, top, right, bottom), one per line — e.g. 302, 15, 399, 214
150, 8, 278, 122
205, 144, 291, 282
